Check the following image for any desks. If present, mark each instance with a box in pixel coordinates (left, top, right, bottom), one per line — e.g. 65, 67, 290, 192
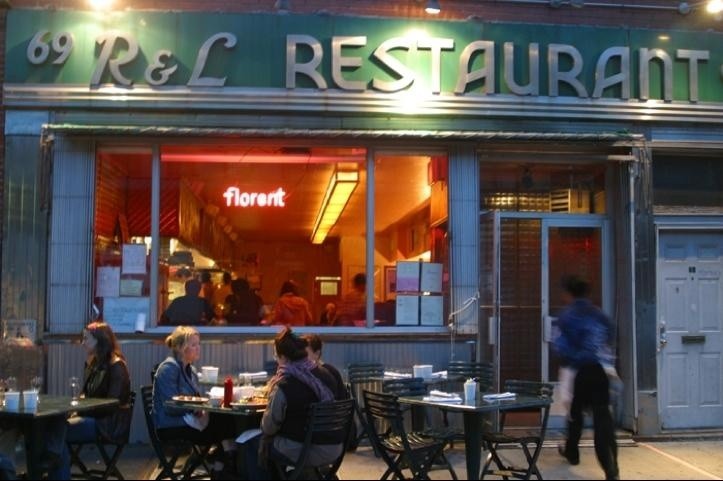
4, 391, 120, 476
397, 388, 551, 480
166, 384, 275, 478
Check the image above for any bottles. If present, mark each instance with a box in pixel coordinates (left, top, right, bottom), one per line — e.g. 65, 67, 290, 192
70, 378, 78, 401
222, 375, 233, 409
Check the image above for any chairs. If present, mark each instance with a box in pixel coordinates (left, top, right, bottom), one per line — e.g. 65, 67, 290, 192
278, 399, 354, 480
140, 383, 200, 481
361, 386, 443, 481
440, 361, 497, 450
383, 377, 464, 480
479, 380, 559, 480
348, 364, 386, 396
69, 387, 138, 479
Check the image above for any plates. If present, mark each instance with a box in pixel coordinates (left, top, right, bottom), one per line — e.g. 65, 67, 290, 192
229, 403, 266, 410
171, 396, 208, 404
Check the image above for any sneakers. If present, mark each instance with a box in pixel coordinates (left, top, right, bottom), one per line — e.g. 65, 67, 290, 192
559, 446, 578, 464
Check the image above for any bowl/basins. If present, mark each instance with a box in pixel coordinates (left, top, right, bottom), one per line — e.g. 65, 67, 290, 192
209, 397, 223, 408
239, 387, 254, 399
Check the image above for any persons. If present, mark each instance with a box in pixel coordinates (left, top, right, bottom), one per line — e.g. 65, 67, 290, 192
299, 331, 359, 453
160, 268, 381, 327
256, 321, 345, 478
547, 260, 626, 480
147, 323, 235, 479
41, 319, 131, 479
0, 448, 21, 481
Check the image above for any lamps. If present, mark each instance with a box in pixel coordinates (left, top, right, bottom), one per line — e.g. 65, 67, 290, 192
307, 159, 361, 245
423, 0, 441, 14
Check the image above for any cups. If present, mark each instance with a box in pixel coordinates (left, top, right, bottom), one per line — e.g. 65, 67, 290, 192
22, 391, 37, 411
200, 365, 218, 383
4, 392, 19, 412
5, 377, 17, 391
411, 365, 432, 380
30, 376, 41, 403
464, 383, 475, 401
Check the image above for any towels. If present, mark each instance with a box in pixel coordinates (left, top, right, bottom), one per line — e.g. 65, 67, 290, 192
382, 370, 412, 379
432, 370, 447, 378
421, 389, 462, 404
484, 391, 517, 400
